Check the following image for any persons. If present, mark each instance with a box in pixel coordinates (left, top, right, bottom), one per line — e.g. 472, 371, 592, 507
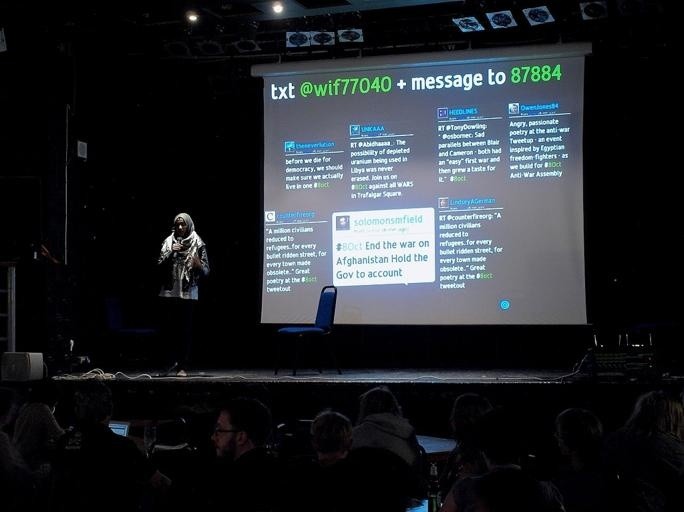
155, 204, 210, 333
338, 217, 348, 229
440, 200, 448, 207
0, 377, 684, 512
511, 104, 518, 114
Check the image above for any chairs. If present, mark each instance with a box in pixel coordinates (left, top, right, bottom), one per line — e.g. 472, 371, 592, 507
272, 285, 342, 376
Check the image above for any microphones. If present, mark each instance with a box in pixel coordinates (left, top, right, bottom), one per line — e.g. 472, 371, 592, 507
174, 236, 182, 258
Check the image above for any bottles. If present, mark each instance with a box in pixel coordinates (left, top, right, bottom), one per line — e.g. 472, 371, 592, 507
427, 462, 442, 512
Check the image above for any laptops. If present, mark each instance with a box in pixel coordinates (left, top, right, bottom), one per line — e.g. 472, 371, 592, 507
107, 419, 130, 436
